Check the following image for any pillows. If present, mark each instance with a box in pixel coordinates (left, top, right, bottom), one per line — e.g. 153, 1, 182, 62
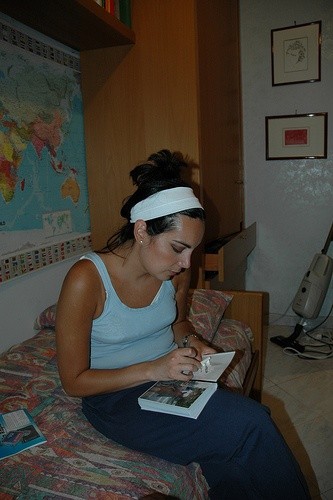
34, 290, 233, 346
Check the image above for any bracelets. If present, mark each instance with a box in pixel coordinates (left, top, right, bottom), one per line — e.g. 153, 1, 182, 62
183, 334, 201, 348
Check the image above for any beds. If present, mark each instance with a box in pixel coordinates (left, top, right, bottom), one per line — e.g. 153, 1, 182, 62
0, 290, 264, 500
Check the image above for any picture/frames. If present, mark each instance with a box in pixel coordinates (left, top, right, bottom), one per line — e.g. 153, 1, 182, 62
271, 20, 321, 86
265, 113, 327, 160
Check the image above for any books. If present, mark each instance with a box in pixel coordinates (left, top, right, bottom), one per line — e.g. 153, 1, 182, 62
0, 408, 47, 459
137, 351, 236, 420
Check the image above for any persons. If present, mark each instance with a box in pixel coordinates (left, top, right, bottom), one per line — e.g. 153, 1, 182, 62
54, 150, 312, 500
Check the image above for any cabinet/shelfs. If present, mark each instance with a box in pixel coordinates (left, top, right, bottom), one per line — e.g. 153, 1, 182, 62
0, 0, 256, 289
